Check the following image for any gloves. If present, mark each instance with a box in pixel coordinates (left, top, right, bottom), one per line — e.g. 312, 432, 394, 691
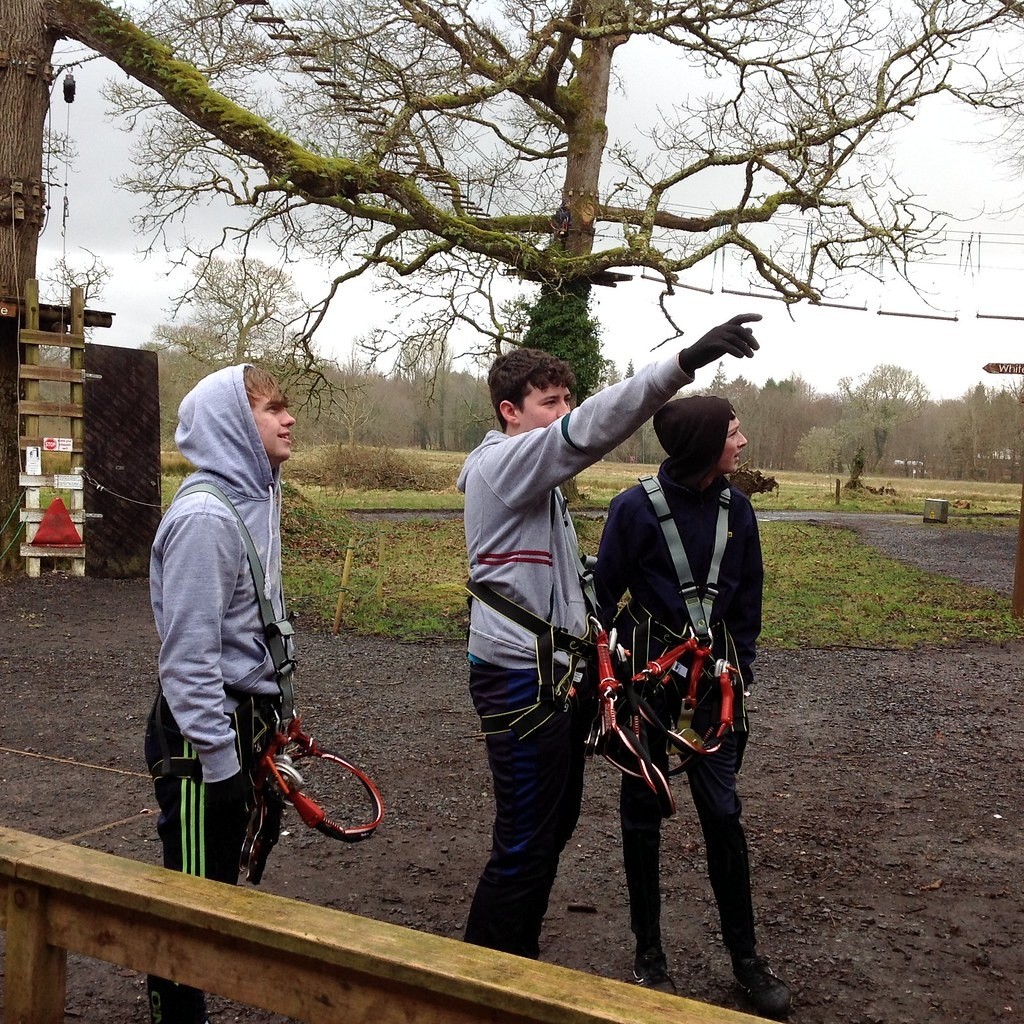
207, 770, 258, 845
679, 312, 763, 375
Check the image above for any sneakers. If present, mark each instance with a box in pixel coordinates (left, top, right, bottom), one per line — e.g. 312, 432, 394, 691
632, 951, 675, 997
733, 954, 792, 1010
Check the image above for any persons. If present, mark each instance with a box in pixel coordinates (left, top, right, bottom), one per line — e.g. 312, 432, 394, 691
144, 362, 297, 1024
457, 311, 793, 1015
